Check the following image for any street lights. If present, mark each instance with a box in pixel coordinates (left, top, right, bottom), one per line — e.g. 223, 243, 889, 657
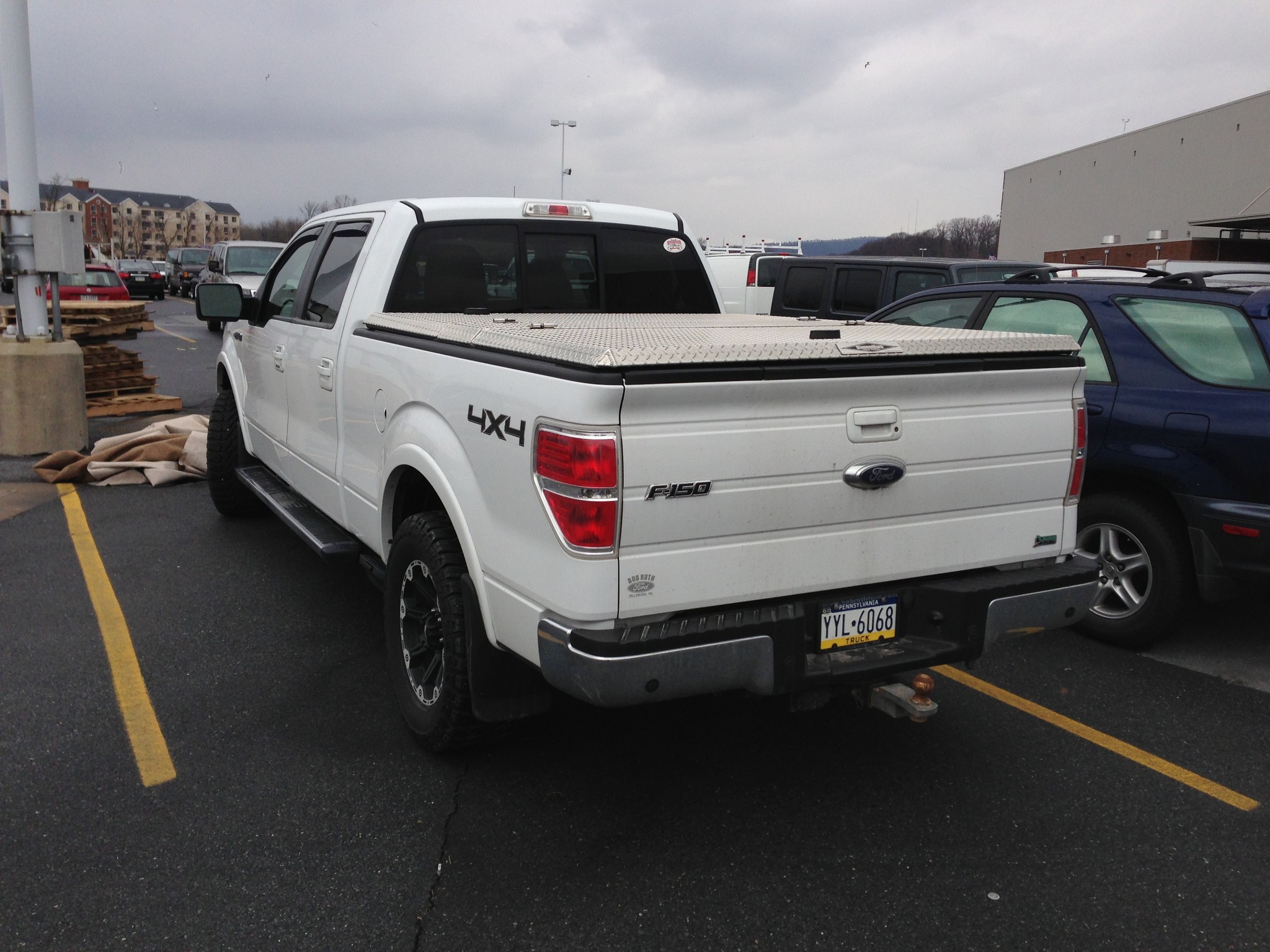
550, 118, 577, 200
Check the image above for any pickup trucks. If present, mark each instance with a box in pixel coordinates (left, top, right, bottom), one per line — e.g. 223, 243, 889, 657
191, 196, 1108, 763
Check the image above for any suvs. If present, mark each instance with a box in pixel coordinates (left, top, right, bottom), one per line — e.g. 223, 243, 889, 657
844, 281, 1270, 655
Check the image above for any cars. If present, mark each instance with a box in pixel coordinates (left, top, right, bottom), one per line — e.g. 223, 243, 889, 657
700, 244, 1269, 321
43, 236, 289, 338
